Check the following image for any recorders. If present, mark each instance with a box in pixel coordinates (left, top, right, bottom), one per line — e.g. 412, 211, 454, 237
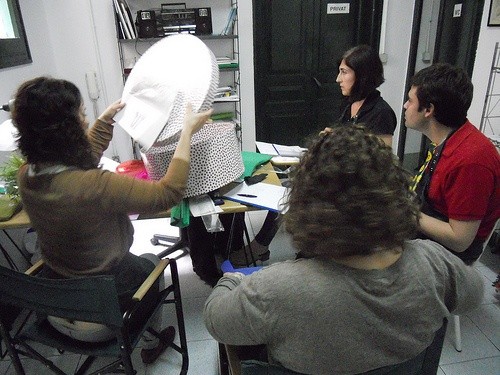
136, 7, 213, 38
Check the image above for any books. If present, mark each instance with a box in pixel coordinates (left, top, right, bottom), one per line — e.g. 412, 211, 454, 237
115, 0, 137, 40
255, 140, 311, 166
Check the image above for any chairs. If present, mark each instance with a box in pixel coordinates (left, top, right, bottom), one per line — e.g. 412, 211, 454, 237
218, 316, 448, 375
0, 258, 189, 375
451, 217, 500, 352
148, 223, 191, 263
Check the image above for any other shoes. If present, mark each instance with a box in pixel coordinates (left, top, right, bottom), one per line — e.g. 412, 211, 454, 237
229, 247, 271, 266
140, 325, 176, 365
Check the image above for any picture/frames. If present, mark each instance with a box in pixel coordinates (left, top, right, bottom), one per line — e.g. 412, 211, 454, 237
487, 0, 500, 27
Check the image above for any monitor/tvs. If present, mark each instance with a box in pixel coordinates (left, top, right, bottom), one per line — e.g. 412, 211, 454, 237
0, 0, 32, 69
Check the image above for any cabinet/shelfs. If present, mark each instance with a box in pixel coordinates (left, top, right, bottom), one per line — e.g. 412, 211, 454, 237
478, 41, 500, 149
113, 0, 242, 161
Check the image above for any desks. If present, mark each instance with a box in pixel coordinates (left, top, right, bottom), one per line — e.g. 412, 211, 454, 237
0, 160, 285, 268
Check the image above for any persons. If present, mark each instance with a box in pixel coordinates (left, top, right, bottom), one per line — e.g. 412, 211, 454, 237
230, 44, 397, 266
202, 124, 485, 375
404, 64, 500, 265
12, 76, 214, 363
